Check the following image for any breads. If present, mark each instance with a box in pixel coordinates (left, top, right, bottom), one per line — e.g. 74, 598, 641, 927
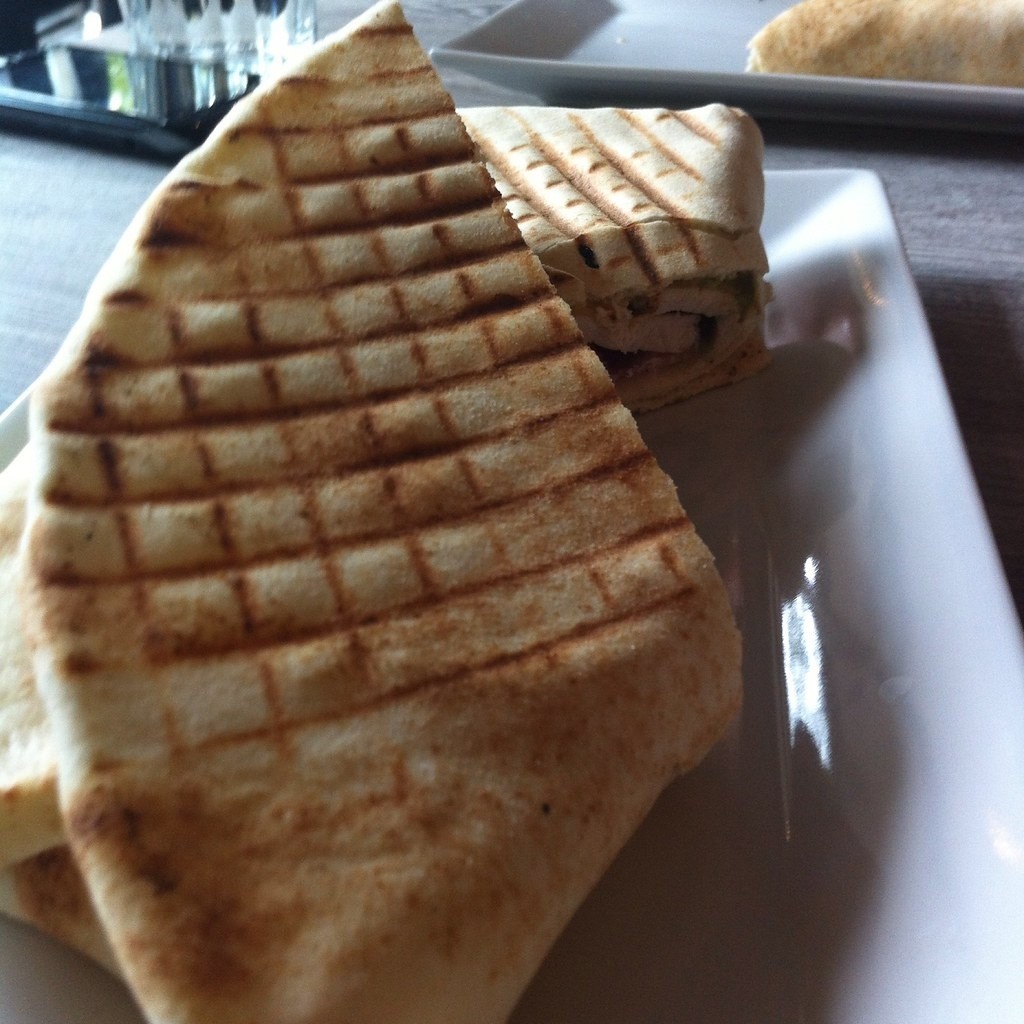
744, 0, 1024, 91
455, 102, 774, 411
0, 0, 742, 1024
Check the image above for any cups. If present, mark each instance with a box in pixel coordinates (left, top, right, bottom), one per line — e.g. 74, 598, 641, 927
117, 0, 316, 76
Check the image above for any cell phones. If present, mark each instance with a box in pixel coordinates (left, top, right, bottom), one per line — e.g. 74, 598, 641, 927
0, 44, 261, 165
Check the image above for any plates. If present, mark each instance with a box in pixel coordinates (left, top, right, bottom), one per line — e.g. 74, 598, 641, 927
0, 168, 1024, 1022
427, 0, 1024, 113
